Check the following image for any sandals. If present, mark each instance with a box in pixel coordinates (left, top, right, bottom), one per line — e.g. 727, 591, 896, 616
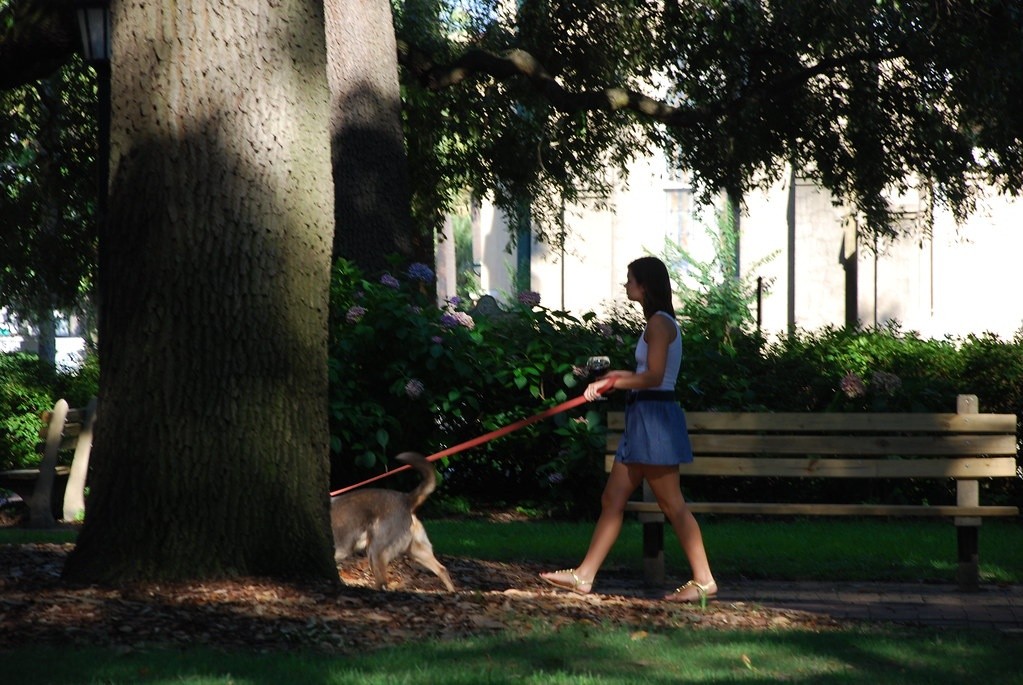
539, 568, 594, 595
664, 580, 717, 601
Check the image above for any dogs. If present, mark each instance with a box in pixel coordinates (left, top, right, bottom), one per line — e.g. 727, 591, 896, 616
330, 451, 461, 596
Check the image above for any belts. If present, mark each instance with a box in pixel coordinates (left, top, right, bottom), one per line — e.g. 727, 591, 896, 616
630, 390, 675, 401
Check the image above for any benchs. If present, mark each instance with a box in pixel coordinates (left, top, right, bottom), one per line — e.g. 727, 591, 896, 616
604, 395, 1019, 588
1, 394, 98, 525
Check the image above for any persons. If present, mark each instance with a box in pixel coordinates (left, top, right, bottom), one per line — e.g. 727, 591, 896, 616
538, 257, 717, 600
